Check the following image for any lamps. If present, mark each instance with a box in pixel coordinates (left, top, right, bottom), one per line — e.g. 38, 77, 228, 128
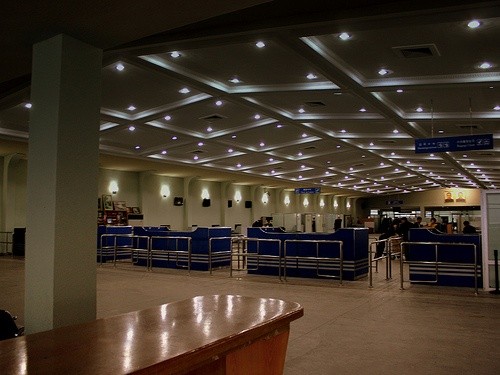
163, 194, 166, 198
112, 191, 117, 195
236, 199, 240, 202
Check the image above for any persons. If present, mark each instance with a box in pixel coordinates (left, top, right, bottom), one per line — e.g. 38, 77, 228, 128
252, 217, 273, 227
118, 212, 128, 226
349, 217, 353, 225
445, 192, 465, 203
312, 218, 315, 231
373, 214, 423, 261
334, 217, 342, 231
463, 221, 476, 234
356, 217, 360, 224
427, 218, 438, 228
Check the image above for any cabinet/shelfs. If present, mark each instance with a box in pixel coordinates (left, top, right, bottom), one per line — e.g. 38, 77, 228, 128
97, 210, 129, 226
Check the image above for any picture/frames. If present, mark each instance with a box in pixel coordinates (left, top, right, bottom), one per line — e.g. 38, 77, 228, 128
102, 194, 140, 214
